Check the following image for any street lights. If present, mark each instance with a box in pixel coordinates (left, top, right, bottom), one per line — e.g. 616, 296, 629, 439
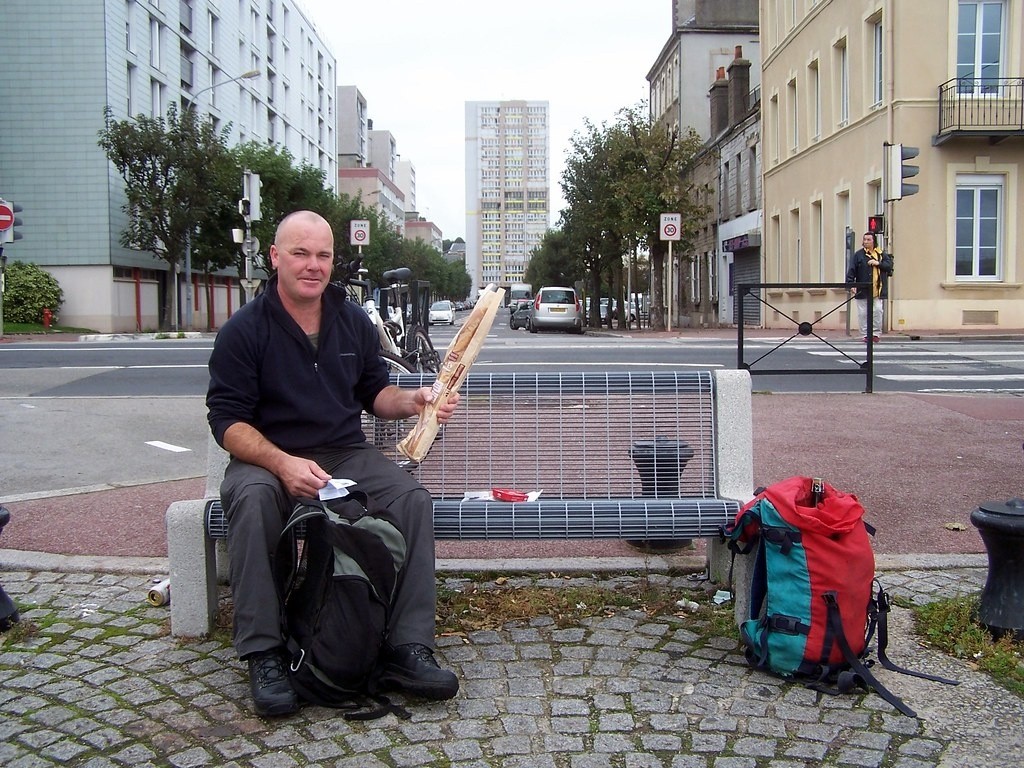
186, 70, 260, 333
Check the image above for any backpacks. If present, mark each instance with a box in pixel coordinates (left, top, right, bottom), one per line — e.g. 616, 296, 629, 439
279, 488, 408, 707
717, 473, 875, 683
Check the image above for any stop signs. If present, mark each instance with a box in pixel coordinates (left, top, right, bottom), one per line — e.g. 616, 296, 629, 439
0, 204, 13, 233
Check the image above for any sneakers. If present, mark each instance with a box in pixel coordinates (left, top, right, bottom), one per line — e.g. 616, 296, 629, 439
372, 642, 459, 701
247, 648, 298, 717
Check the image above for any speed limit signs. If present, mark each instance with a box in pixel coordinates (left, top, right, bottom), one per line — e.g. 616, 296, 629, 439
665, 224, 677, 236
355, 231, 367, 241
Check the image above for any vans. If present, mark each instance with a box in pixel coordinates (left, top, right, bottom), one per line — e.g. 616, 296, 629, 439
529, 285, 581, 334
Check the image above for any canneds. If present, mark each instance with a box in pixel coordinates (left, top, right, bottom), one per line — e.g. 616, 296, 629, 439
147, 577, 170, 607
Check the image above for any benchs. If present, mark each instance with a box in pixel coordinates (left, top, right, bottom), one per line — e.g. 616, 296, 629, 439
164, 359, 762, 640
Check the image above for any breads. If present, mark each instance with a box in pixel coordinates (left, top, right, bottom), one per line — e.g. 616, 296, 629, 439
404, 304, 489, 456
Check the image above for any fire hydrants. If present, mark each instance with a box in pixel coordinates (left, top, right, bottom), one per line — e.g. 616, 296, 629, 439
44, 309, 52, 326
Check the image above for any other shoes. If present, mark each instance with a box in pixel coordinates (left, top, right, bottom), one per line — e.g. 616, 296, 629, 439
873, 335, 880, 342
864, 337, 868, 343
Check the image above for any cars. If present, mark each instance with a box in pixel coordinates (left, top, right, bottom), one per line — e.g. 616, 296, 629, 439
429, 300, 456, 325
579, 297, 639, 323
510, 301, 536, 330
452, 301, 476, 311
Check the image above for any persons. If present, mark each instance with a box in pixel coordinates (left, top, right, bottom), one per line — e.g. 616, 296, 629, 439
846, 232, 892, 342
206, 210, 460, 715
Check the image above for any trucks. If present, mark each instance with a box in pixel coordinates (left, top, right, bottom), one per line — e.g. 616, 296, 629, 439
510, 284, 532, 315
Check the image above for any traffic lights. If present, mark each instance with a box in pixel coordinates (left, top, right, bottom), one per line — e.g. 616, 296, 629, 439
13, 202, 24, 240
891, 144, 919, 200
249, 174, 262, 222
868, 216, 884, 233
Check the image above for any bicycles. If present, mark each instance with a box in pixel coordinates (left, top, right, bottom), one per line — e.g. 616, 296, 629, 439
332, 254, 453, 442
